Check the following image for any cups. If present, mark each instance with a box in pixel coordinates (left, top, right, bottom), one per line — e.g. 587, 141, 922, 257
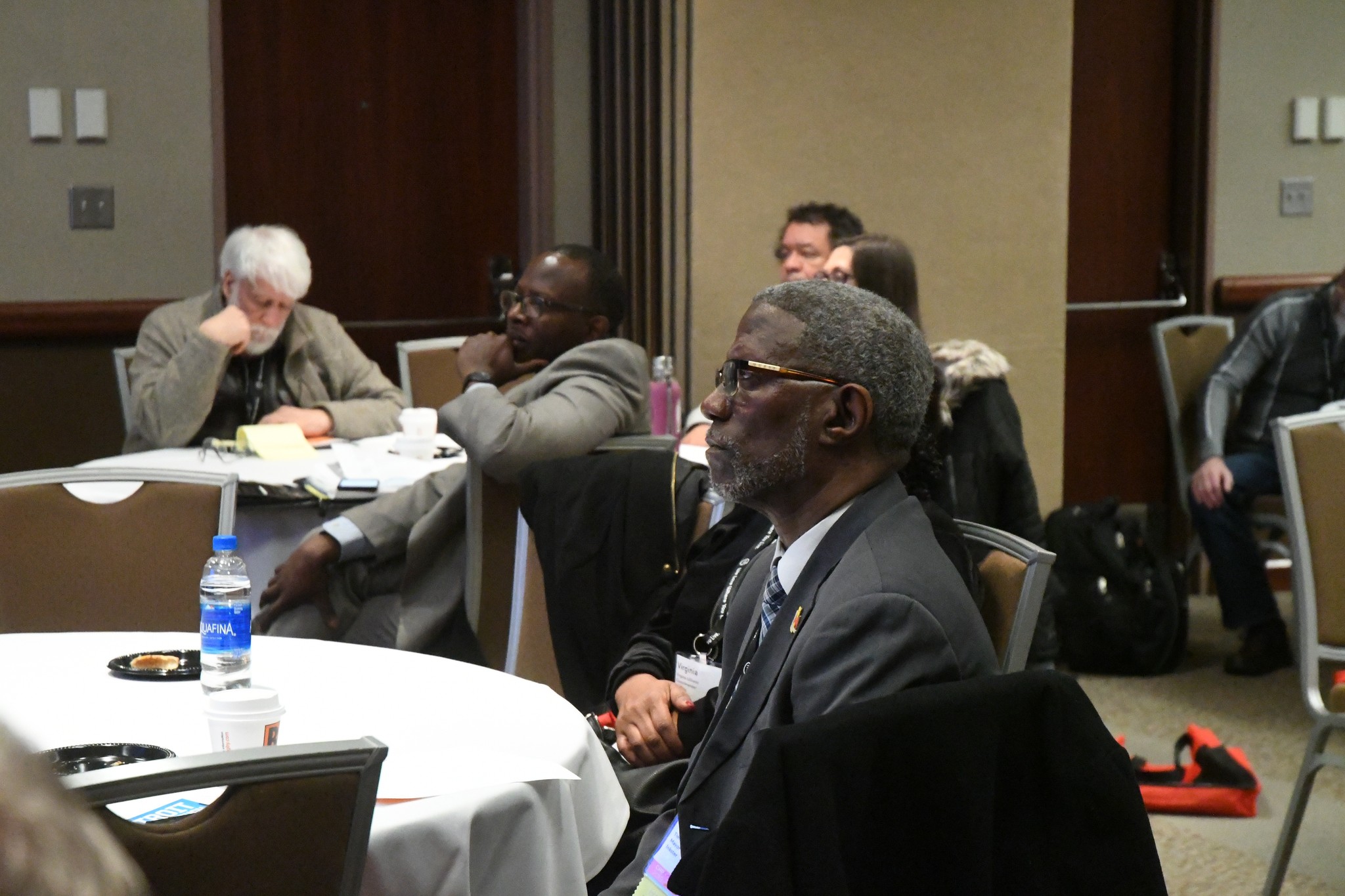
205, 688, 282, 752
398, 407, 438, 462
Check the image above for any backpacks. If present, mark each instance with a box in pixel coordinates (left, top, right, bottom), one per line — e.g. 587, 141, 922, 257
1047, 504, 1189, 677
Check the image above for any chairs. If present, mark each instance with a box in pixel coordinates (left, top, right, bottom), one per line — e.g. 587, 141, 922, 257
62, 735, 388, 896
1153, 315, 1291, 576
1259, 411, 1345, 895
0, 465, 240, 632
504, 487, 729, 697
464, 433, 679, 670
396, 334, 533, 408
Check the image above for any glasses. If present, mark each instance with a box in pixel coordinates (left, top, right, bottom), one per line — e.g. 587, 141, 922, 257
814, 269, 855, 285
498, 290, 588, 317
714, 360, 845, 396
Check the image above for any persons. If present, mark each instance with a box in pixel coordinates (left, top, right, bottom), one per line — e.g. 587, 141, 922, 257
680, 201, 867, 447
1187, 268, 1345, 672
585, 281, 999, 896
813, 232, 921, 336
120, 224, 411, 457
245, 242, 654, 664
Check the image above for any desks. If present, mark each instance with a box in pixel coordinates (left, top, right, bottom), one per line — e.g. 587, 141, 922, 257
64, 427, 468, 623
0, 631, 632, 896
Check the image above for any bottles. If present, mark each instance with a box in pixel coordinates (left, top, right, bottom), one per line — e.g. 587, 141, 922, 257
650, 355, 682, 452
199, 535, 252, 695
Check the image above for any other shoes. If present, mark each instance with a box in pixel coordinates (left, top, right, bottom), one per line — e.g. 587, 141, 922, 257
1224, 618, 1295, 678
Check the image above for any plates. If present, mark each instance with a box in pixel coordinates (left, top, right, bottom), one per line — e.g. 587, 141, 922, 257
107, 650, 201, 678
33, 743, 177, 778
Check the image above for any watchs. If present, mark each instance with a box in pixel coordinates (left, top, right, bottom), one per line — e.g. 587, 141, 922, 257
462, 372, 492, 394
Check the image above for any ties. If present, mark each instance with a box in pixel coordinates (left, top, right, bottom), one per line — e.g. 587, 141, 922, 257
757, 564, 788, 648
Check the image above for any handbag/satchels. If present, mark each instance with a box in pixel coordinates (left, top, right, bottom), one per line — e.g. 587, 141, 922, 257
1120, 726, 1262, 818
